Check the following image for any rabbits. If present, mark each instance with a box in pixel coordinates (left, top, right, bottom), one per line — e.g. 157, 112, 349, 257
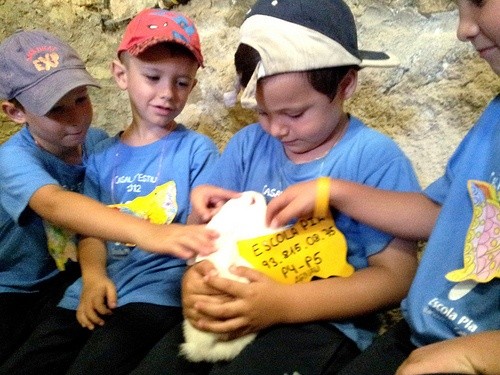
177, 190, 295, 362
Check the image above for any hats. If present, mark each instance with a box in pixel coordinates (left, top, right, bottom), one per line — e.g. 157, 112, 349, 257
223, 0, 400, 109
0, 29, 102, 117
118, 8, 204, 68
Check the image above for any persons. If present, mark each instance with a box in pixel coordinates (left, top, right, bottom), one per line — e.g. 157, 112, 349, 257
133, 0, 421, 375
265, 0, 499, 375
0, 8, 240, 375
0, 30, 220, 366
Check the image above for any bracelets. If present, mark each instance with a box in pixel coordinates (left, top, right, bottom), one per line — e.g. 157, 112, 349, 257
312, 177, 330, 222
